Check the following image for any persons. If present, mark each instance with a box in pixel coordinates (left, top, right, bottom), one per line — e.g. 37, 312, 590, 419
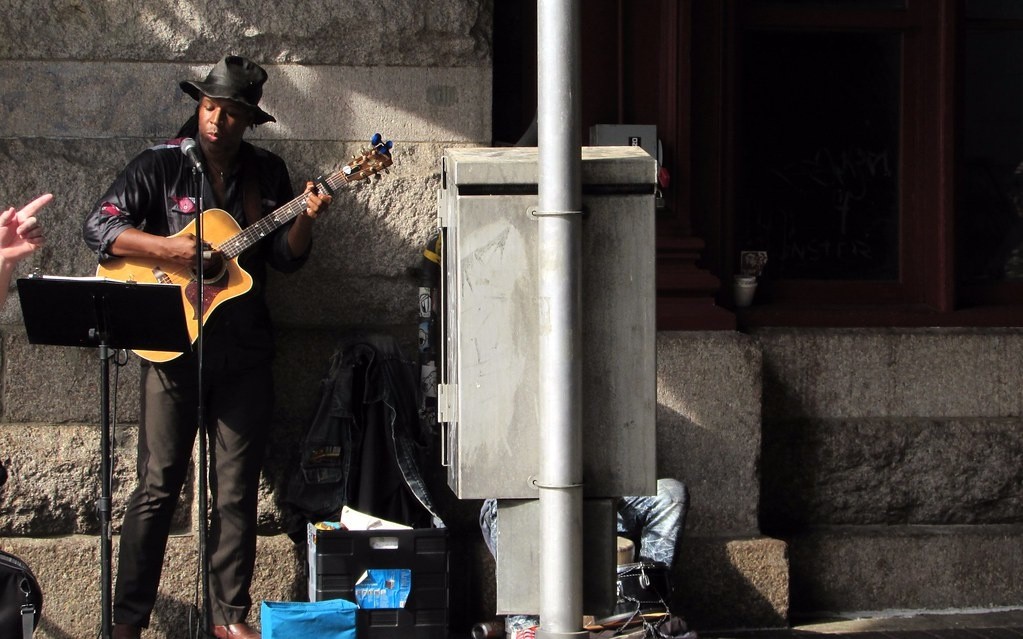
83, 55, 332, 639
480, 478, 690, 639
0, 194, 53, 311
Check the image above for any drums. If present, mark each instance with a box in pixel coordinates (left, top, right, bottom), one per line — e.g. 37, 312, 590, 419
591, 559, 677, 627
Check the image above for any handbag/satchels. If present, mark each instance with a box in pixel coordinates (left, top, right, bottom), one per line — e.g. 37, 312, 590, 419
0, 550, 43, 639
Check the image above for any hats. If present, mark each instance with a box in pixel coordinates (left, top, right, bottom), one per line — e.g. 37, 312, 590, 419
180, 56, 277, 126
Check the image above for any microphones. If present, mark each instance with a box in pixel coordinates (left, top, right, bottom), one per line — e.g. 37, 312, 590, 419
180, 138, 205, 173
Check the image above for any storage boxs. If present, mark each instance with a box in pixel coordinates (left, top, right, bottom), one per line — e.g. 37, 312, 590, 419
309, 519, 454, 639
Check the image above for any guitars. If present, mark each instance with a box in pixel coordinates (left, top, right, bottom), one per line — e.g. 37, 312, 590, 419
93, 144, 395, 368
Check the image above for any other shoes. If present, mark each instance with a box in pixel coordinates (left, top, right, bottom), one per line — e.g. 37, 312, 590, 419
510, 625, 539, 639
208, 623, 262, 639
111, 624, 142, 639
